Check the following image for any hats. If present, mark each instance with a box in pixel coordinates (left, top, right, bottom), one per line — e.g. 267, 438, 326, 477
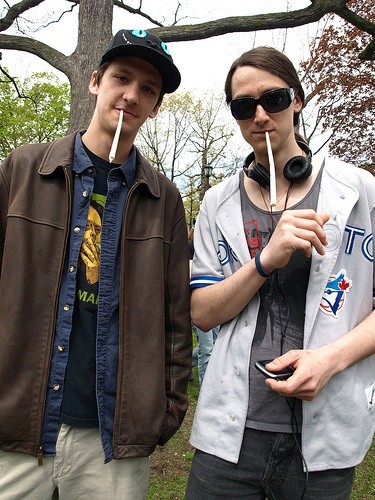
100, 30, 181, 93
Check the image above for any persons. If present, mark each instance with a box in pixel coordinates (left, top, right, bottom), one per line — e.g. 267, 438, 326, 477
187, 217, 220, 388
0, 29, 193, 500
184, 46, 375, 500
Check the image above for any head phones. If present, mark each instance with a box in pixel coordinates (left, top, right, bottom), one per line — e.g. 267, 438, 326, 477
242, 140, 313, 190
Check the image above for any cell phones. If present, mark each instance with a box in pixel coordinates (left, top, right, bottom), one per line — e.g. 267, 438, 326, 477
255, 359, 296, 380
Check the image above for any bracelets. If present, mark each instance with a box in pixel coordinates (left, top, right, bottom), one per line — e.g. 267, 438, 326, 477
255, 250, 275, 278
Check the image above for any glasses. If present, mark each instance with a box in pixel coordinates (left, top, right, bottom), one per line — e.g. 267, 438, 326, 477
230, 87, 296, 120
